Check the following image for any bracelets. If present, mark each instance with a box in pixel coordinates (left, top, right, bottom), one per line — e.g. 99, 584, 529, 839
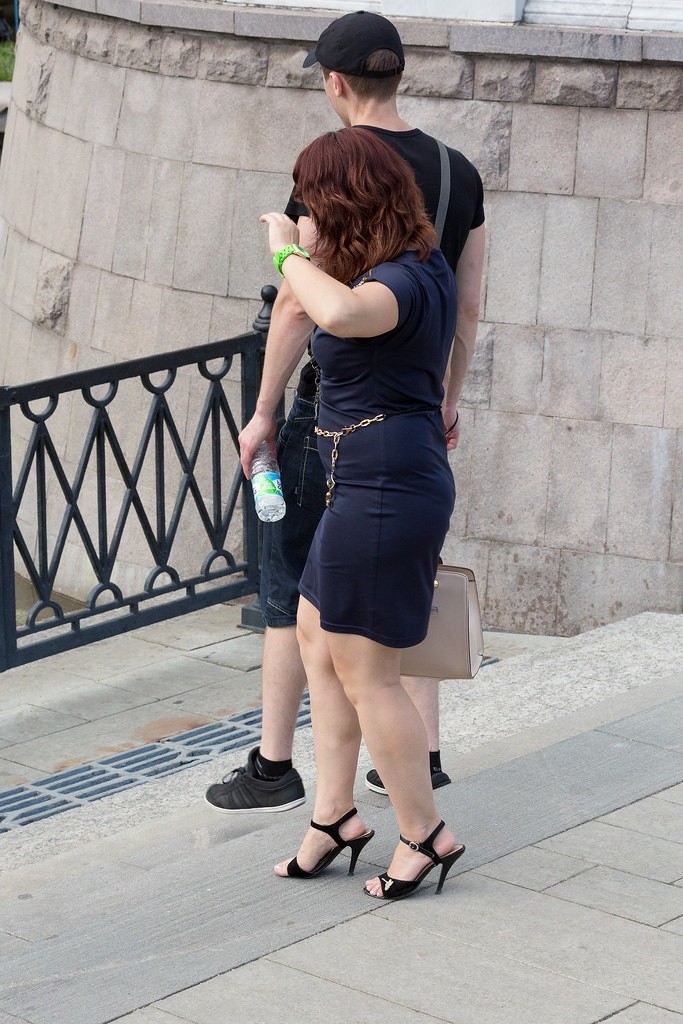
444, 410, 459, 435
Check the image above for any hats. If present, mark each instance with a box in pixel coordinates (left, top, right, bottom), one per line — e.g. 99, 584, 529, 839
302, 10, 406, 78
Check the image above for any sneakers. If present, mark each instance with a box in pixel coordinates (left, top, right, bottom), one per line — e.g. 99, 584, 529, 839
203, 745, 306, 813
365, 767, 451, 795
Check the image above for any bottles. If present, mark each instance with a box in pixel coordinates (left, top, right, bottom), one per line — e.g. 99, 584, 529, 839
249, 439, 286, 523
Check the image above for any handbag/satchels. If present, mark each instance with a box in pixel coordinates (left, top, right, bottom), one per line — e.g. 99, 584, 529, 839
399, 553, 485, 679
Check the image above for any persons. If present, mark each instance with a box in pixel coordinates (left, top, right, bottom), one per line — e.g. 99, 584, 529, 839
259, 128, 467, 901
204, 10, 487, 815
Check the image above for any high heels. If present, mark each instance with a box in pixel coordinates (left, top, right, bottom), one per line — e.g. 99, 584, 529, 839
279, 807, 375, 879
363, 820, 466, 899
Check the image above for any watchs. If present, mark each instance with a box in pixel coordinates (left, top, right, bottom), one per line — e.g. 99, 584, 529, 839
273, 244, 312, 277
